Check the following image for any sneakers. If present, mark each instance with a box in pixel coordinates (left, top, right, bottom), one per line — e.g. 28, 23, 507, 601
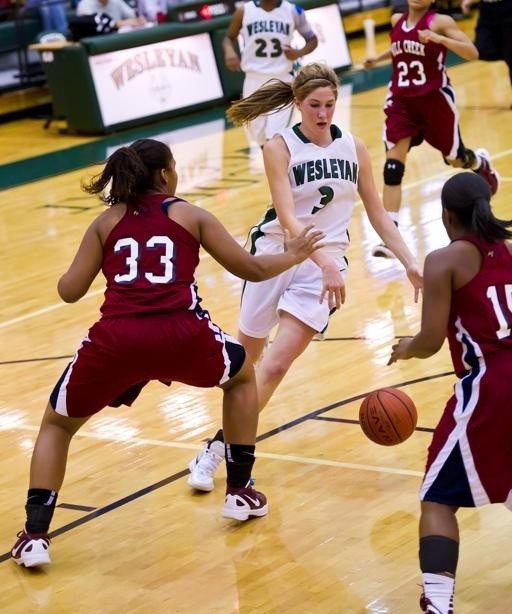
373, 244, 396, 258
188, 440, 225, 492
11, 529, 51, 567
473, 149, 499, 195
223, 480, 268, 521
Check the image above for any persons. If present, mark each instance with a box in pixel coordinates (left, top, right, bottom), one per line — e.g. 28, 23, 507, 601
386, 171, 512, 614
221, 1, 319, 153
186, 63, 426, 491
10, 0, 70, 39
10, 137, 326, 568
458, 0, 512, 109
68, 1, 146, 43
362, 1, 498, 256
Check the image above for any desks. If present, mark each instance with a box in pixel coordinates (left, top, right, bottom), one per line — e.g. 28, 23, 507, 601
27, 0, 355, 135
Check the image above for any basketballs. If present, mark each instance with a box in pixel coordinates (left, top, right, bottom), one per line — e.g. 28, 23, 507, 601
359, 389, 417, 446
